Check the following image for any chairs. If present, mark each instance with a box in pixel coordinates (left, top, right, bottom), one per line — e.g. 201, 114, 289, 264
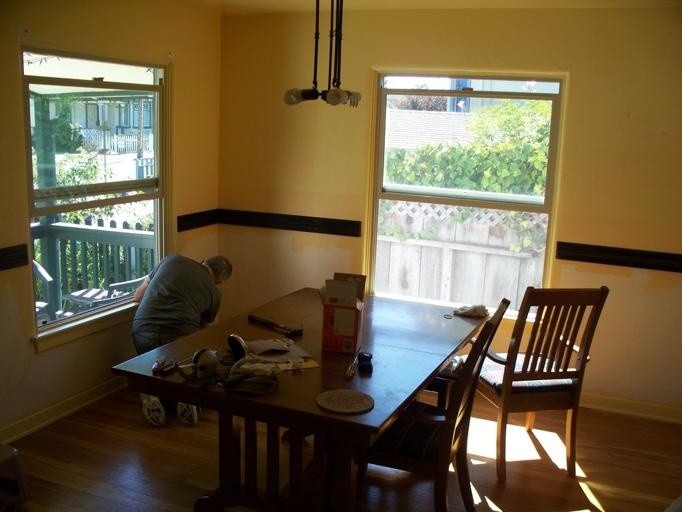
193, 397, 324, 512
462, 286, 609, 483
324, 298, 510, 512
62, 273, 149, 311
32, 260, 55, 323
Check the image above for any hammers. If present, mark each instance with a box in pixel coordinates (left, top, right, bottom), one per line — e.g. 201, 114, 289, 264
248, 313, 304, 338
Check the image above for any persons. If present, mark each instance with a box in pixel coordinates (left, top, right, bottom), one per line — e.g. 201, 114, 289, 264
132, 255, 233, 427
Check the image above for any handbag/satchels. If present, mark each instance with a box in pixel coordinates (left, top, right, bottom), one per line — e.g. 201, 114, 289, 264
224, 357, 279, 394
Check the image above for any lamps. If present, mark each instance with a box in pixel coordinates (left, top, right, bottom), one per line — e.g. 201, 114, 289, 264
284, 0, 361, 107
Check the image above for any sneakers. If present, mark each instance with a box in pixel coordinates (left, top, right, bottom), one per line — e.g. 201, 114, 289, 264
140, 392, 165, 426
177, 401, 197, 426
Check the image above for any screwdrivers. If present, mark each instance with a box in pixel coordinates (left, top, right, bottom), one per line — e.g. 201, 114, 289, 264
345, 346, 362, 379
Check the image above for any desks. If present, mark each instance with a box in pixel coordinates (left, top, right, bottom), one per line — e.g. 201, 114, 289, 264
113, 287, 488, 512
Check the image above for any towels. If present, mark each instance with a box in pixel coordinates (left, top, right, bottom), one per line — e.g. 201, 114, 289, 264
453, 305, 489, 317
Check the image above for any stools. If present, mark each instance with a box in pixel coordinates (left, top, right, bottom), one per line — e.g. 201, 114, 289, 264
0, 444, 32, 499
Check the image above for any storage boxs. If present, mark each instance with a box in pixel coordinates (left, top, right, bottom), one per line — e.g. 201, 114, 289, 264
319, 272, 366, 354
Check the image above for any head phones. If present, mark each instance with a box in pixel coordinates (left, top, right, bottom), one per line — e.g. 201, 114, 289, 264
176, 333, 248, 379
